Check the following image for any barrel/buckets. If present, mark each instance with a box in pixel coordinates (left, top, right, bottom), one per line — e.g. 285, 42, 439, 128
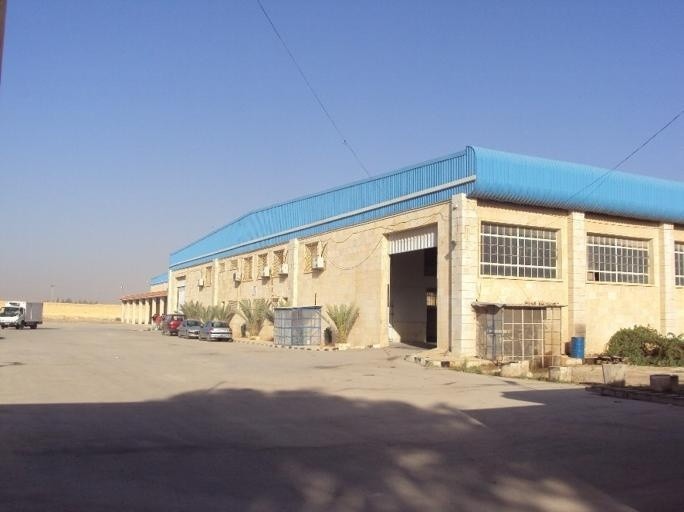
572, 337, 585, 360
273, 307, 296, 345
290, 307, 321, 346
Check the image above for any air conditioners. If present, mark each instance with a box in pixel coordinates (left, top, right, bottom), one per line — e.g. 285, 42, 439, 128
197, 278, 203, 287
232, 256, 325, 283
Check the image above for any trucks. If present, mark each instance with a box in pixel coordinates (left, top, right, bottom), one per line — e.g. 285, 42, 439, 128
0, 301, 43, 329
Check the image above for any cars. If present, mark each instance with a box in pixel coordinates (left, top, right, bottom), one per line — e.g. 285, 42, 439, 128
163, 314, 232, 342
